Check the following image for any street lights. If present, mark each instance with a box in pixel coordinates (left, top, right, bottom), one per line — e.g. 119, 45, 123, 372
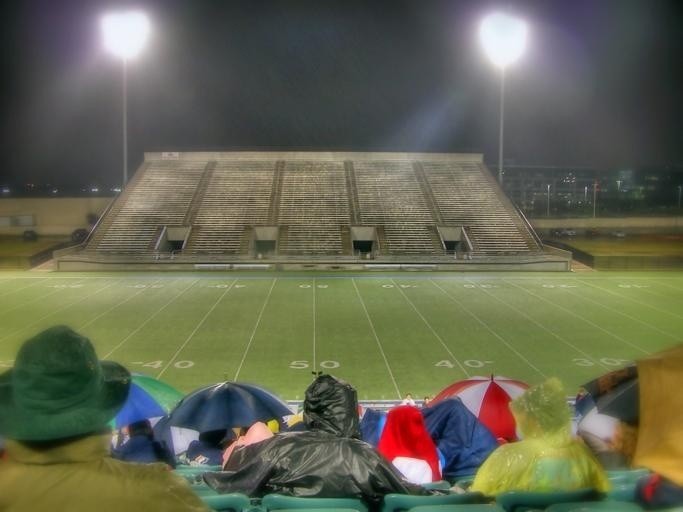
95, 8, 153, 186
478, 11, 531, 187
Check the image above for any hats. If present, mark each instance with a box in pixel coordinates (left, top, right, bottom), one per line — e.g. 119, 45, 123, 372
1, 325, 132, 444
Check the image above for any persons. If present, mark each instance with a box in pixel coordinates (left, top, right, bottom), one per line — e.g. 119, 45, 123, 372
2, 327, 682, 512
